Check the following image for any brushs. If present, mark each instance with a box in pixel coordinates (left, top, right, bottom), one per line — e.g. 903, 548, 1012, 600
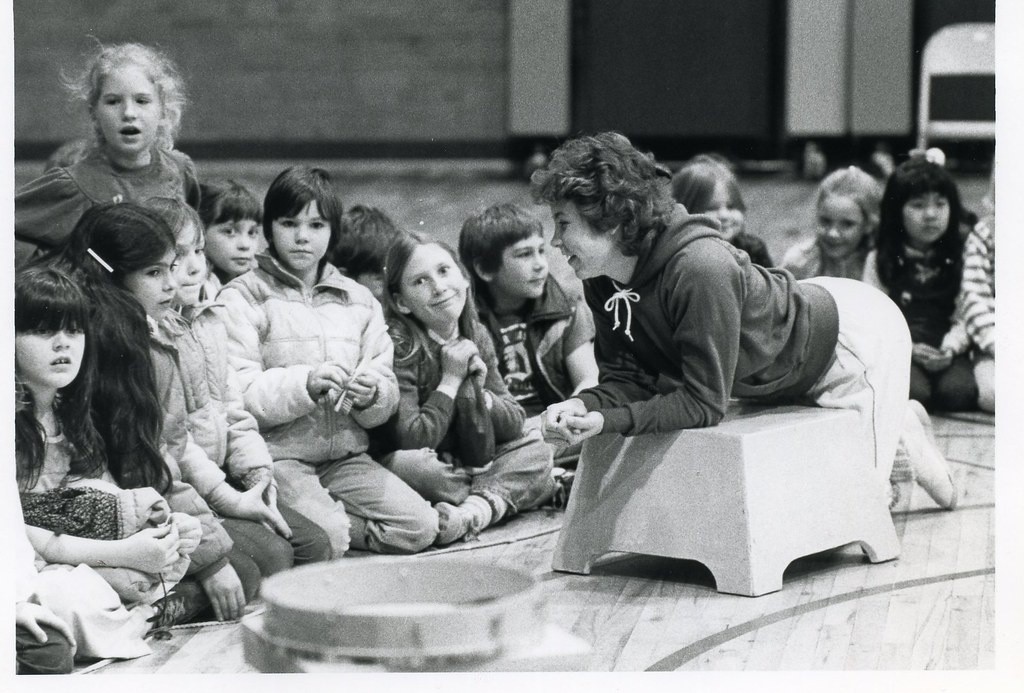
334, 360, 363, 419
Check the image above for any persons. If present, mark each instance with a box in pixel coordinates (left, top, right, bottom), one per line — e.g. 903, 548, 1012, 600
779, 165, 887, 295
873, 157, 995, 414
15, 141, 599, 674
670, 156, 772, 268
14, 42, 201, 270
540, 131, 954, 509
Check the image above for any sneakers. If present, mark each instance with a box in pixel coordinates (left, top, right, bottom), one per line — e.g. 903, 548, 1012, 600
150, 583, 206, 640
900, 400, 955, 509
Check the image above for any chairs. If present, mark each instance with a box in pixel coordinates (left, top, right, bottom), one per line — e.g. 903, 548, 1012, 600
916, 23, 996, 153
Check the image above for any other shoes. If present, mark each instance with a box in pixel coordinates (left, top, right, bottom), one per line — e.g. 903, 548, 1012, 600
434, 500, 481, 544
525, 463, 578, 512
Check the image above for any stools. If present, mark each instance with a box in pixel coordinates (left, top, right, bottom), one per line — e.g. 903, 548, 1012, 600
551, 396, 899, 597
